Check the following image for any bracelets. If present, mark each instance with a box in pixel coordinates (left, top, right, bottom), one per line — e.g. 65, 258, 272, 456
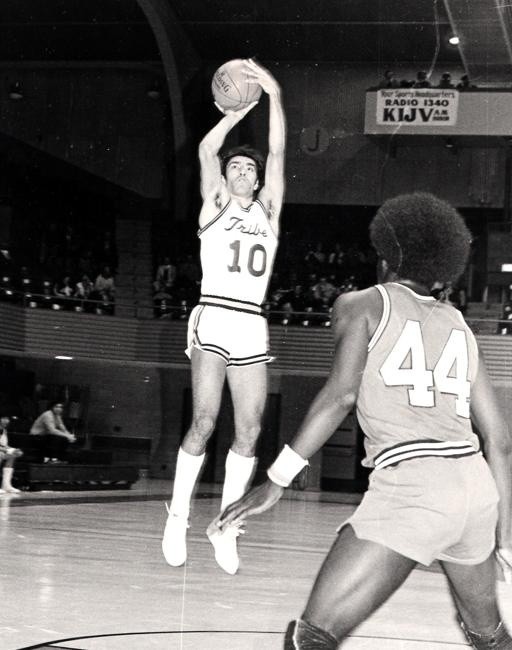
265, 443, 310, 488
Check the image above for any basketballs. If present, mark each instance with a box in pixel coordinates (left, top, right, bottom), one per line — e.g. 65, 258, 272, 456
212, 57, 262, 112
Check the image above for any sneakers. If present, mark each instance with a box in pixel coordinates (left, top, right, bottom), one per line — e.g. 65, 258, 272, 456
161, 513, 187, 566
205, 519, 240, 575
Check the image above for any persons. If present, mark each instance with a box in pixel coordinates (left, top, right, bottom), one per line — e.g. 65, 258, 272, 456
498, 284, 512, 334
1, 222, 115, 311
213, 192, 512, 649
437, 287, 466, 313
29, 402, 79, 463
0, 415, 25, 495
162, 58, 289, 576
379, 69, 478, 91
154, 239, 373, 328
